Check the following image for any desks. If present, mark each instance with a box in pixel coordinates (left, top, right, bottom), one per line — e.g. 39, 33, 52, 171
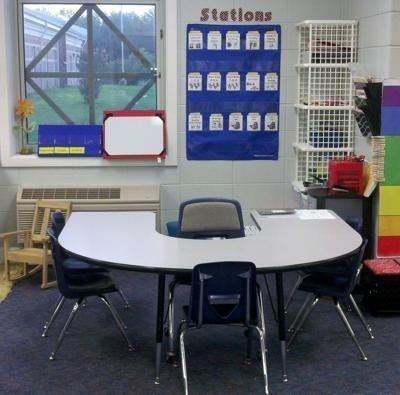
57, 211, 363, 386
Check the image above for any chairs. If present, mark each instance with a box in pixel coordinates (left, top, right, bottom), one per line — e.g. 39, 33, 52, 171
284, 216, 374, 360
41, 212, 135, 360
165, 199, 270, 395
0, 202, 71, 289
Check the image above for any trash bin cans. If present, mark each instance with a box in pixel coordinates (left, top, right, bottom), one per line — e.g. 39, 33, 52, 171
363, 257, 400, 318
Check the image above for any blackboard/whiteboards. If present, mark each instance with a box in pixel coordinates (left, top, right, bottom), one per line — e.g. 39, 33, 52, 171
103, 110, 166, 159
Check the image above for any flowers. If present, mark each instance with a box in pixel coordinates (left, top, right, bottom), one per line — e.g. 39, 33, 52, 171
11, 96, 36, 144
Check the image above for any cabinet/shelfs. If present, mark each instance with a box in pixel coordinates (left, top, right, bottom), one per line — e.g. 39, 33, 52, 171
291, 19, 360, 190
